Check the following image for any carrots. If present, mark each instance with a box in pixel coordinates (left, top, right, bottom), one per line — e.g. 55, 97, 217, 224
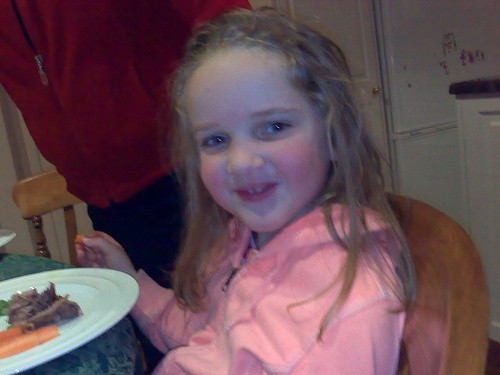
0, 327, 61, 361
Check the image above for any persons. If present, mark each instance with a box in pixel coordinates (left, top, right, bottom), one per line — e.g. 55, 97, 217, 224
0, 0, 254, 371
74, 7, 417, 375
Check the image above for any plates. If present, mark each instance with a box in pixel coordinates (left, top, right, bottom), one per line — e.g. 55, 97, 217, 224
0, 268, 139, 375
0, 229, 16, 247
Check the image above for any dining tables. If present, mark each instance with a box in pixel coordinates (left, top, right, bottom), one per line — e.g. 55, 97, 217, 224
0, 253, 147, 375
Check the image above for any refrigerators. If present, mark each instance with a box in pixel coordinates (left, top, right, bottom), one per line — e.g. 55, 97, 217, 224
374, 0, 500, 227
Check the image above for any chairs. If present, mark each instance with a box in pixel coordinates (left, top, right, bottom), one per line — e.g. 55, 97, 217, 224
382, 192, 490, 375
13, 167, 82, 267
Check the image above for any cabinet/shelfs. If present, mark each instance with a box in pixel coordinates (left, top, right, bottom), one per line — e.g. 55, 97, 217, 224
449, 77, 500, 343
